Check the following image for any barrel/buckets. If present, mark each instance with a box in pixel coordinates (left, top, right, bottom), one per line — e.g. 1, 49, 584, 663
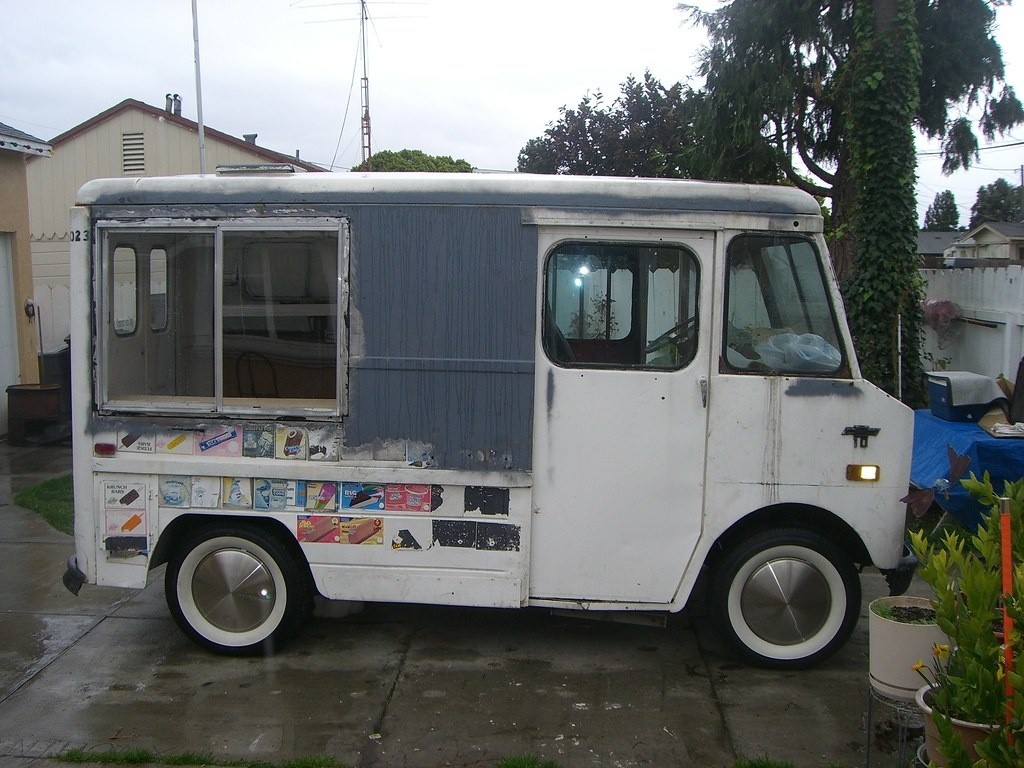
7, 384, 64, 446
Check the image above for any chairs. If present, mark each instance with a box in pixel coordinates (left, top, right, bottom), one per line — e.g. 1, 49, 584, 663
543, 298, 576, 362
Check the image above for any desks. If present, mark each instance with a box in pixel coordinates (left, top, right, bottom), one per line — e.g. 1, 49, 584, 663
908, 410, 1024, 537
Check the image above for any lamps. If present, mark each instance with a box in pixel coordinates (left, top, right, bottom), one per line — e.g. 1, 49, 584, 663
23, 296, 35, 323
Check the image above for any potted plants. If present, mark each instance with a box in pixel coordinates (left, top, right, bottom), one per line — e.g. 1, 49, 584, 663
869, 595, 957, 705
905, 470, 1024, 768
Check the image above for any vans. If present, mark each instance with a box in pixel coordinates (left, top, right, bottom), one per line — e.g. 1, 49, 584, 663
62, 159, 916, 671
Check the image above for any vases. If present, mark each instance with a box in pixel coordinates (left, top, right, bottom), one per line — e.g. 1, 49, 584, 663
917, 743, 929, 768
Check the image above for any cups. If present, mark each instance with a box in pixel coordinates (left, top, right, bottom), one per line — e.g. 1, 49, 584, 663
404, 486, 428, 511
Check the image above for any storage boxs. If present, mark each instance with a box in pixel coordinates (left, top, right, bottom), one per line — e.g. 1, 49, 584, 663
927, 376, 988, 422
